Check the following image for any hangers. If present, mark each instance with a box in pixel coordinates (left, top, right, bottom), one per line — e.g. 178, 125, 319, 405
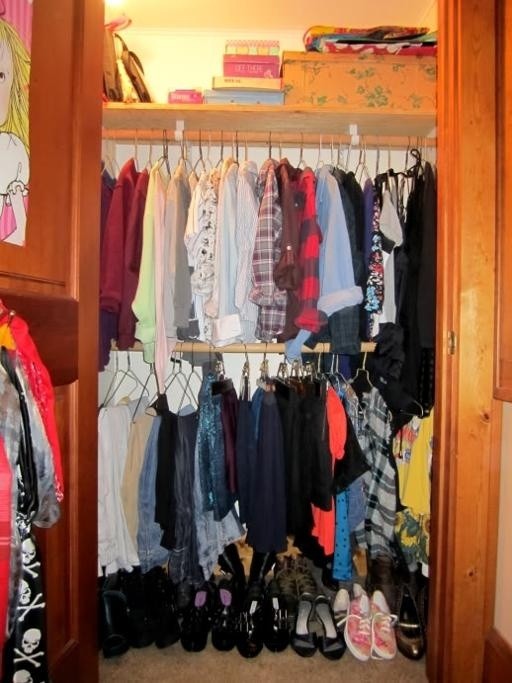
101, 125, 429, 194
98, 338, 424, 425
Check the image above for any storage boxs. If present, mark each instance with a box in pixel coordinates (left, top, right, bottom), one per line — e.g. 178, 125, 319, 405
280, 49, 437, 115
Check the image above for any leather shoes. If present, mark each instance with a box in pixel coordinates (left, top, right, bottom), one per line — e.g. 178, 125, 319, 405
98, 570, 346, 663
395, 579, 431, 660
277, 544, 422, 598
334, 582, 365, 630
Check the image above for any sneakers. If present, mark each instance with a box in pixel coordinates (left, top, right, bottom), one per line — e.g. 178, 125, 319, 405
344, 591, 398, 662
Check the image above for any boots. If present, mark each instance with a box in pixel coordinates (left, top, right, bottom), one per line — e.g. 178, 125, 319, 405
218, 537, 275, 593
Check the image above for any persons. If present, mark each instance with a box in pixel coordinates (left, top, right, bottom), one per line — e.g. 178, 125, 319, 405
0, 19, 31, 246
